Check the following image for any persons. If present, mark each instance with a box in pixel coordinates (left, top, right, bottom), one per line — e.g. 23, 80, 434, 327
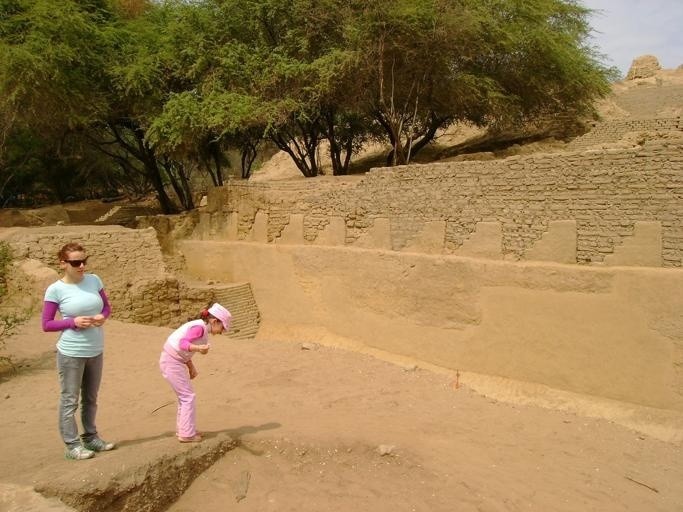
40, 242, 117, 460
158, 303, 232, 443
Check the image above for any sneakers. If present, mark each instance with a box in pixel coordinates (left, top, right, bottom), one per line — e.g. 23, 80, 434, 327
82, 434, 115, 451
176, 431, 203, 443
64, 444, 95, 461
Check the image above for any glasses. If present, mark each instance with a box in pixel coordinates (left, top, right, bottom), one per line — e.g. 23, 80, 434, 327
64, 257, 87, 269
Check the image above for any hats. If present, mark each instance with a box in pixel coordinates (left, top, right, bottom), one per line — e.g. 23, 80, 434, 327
206, 303, 233, 332
